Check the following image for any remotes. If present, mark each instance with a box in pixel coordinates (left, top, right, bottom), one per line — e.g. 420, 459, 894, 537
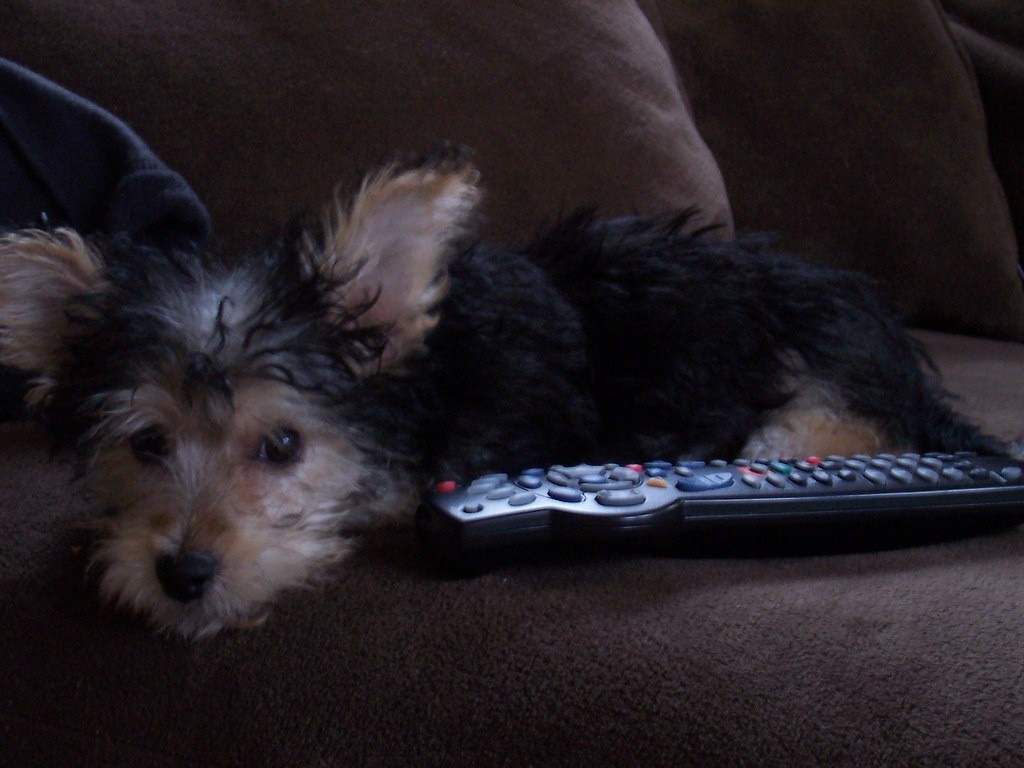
410, 447, 1024, 563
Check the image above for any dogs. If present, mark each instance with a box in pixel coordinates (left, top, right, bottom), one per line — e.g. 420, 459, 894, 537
0, 139, 1024, 646
